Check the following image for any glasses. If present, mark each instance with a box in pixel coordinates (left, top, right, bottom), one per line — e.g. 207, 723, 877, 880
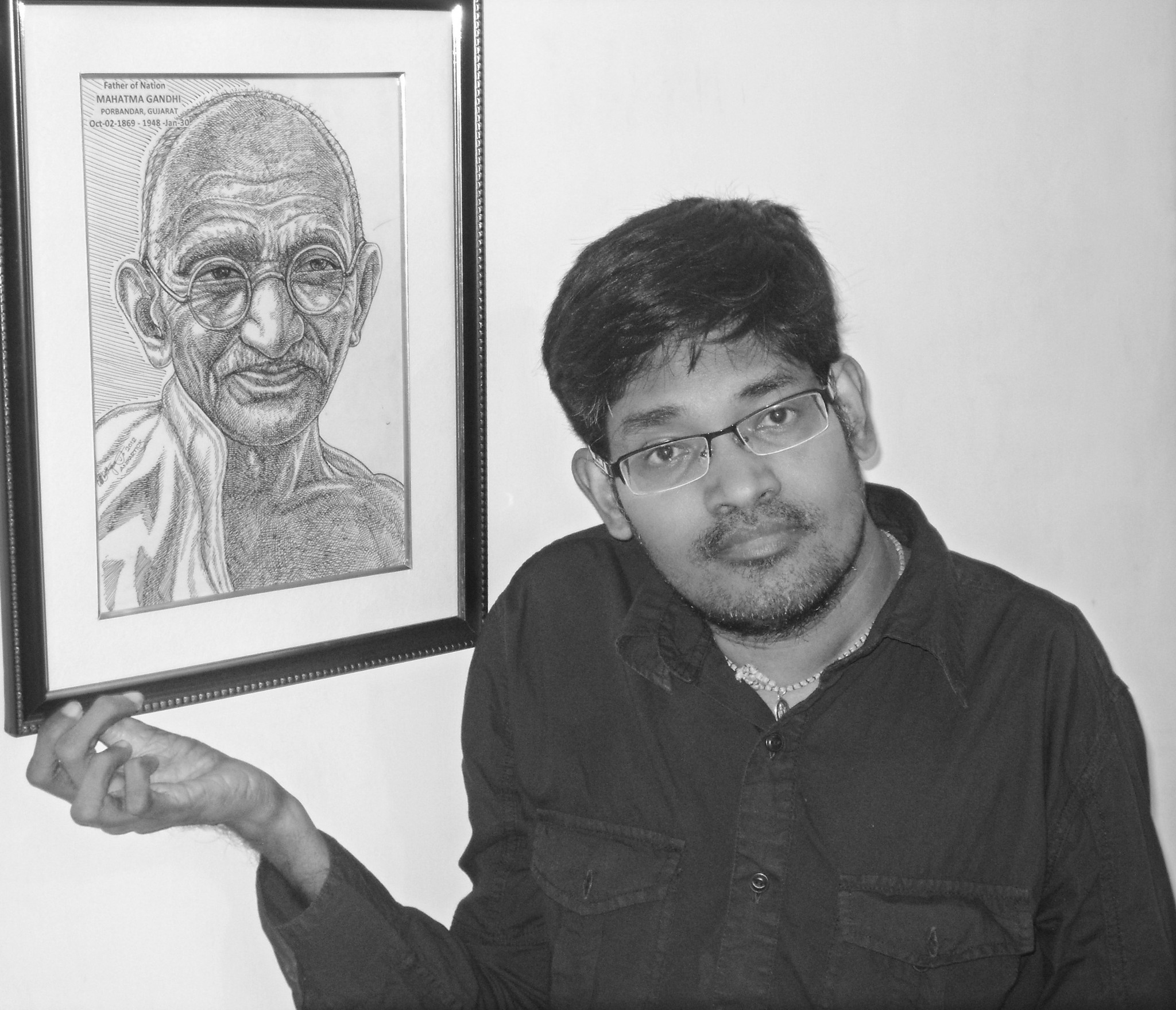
585, 368, 834, 496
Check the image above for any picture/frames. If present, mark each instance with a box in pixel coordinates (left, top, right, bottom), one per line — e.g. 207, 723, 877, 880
0, 0, 488, 738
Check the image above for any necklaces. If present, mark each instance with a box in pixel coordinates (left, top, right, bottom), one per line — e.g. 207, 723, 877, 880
722, 531, 905, 722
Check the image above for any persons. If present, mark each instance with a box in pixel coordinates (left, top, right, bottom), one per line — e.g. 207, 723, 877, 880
27, 200, 1176, 1010
